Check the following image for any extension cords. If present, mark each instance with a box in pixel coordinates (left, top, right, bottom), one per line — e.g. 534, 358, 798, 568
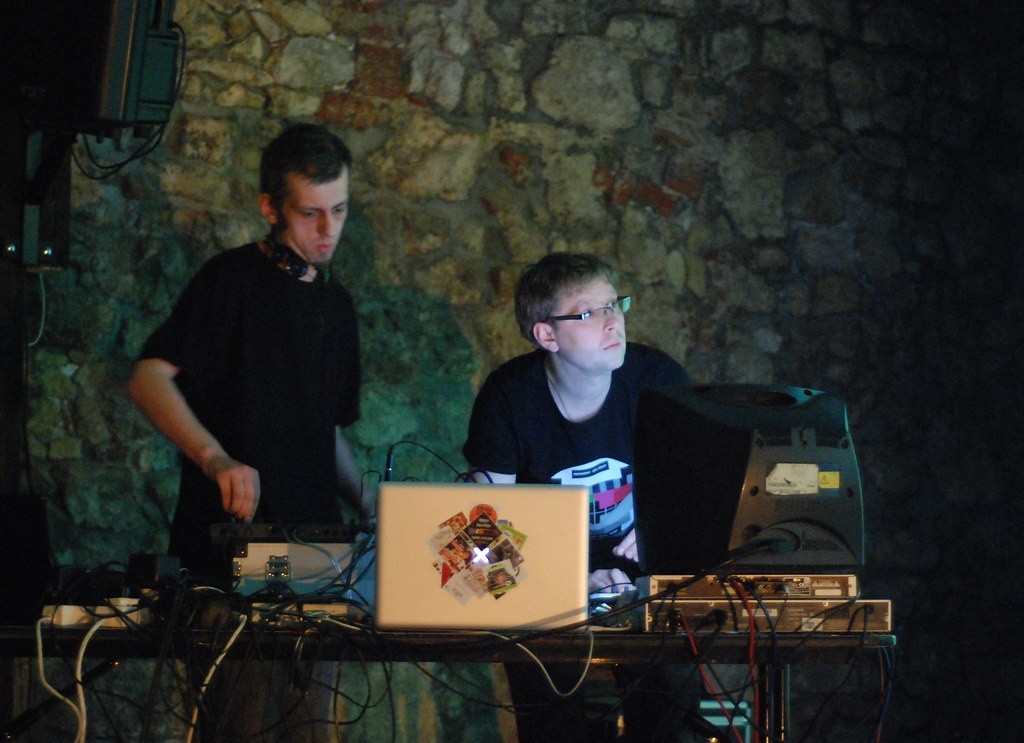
39, 595, 156, 629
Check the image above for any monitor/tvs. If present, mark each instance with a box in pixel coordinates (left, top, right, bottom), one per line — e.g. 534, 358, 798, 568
636, 382, 866, 573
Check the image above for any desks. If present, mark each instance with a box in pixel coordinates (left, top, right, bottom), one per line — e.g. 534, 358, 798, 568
0, 625, 897, 742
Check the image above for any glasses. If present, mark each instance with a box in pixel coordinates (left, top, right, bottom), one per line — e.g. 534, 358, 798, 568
546, 294, 632, 326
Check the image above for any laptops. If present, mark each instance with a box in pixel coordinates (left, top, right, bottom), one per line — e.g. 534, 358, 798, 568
375, 482, 590, 631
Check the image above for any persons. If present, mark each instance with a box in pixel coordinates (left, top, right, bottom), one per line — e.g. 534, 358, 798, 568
467, 247, 740, 741
123, 117, 399, 741
